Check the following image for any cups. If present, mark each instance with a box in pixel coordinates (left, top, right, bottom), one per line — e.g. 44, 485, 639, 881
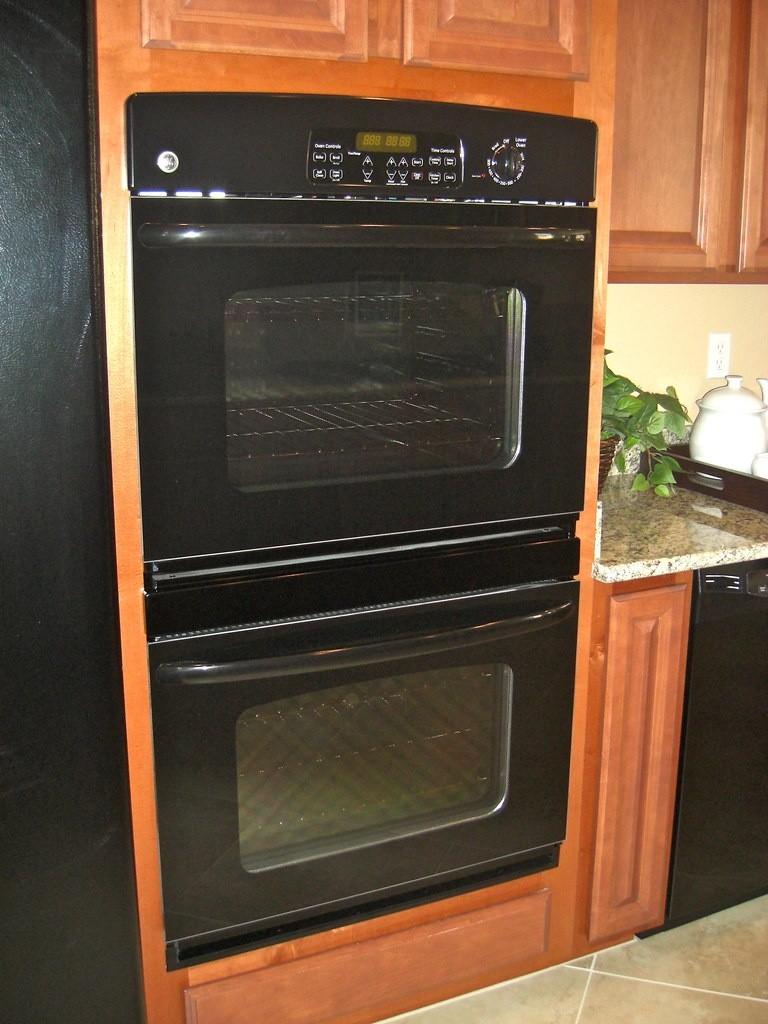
751, 452, 768, 479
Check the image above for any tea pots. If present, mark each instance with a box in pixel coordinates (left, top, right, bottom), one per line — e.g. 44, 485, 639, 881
689, 375, 768, 474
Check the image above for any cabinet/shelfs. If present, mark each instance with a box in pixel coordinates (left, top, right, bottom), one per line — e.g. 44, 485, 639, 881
736, 0, 768, 284
94, 0, 751, 285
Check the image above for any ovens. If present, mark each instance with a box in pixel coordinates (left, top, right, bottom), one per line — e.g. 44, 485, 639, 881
124, 91, 599, 974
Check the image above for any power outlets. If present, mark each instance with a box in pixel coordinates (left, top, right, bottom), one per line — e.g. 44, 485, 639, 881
706, 332, 731, 379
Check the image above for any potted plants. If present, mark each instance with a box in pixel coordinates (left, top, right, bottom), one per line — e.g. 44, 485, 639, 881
598, 347, 695, 500
